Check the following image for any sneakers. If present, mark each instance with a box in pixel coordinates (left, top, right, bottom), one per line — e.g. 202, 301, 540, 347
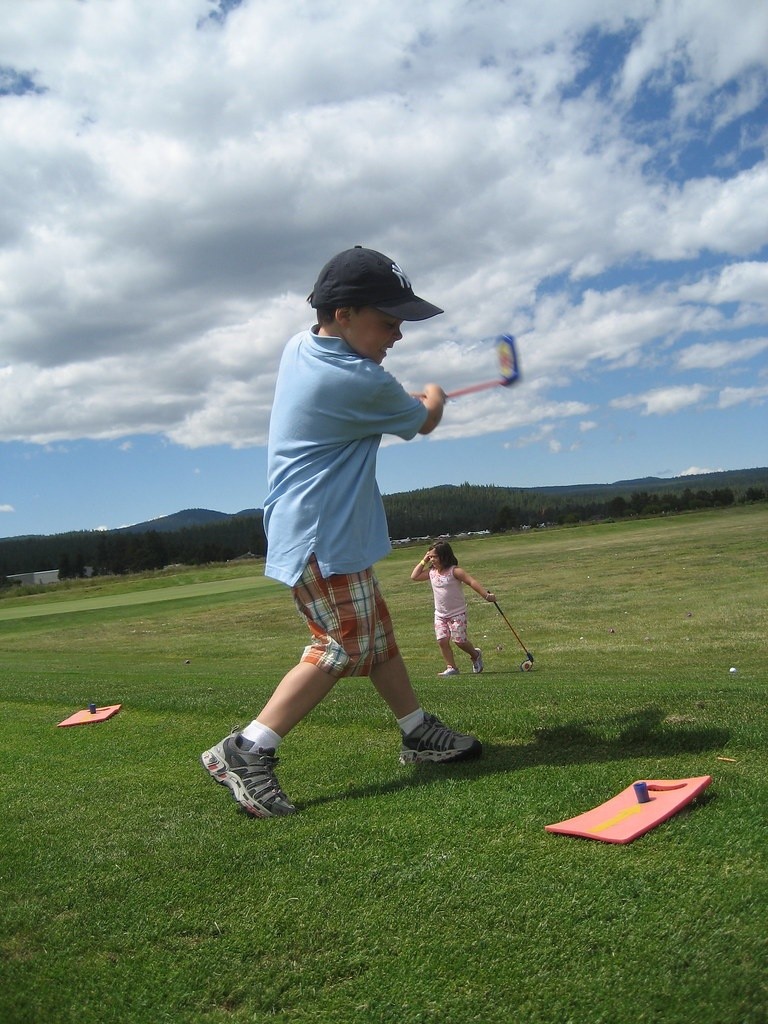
437, 665, 459, 676
399, 712, 483, 765
471, 648, 483, 673
202, 725, 296, 818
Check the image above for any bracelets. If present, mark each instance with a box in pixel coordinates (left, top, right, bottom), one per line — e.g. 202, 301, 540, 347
485, 593, 489, 600
420, 560, 426, 566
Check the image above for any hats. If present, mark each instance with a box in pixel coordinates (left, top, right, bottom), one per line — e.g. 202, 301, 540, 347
307, 245, 445, 320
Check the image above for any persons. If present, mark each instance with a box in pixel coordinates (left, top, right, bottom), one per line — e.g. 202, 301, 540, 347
411, 540, 496, 676
201, 246, 481, 817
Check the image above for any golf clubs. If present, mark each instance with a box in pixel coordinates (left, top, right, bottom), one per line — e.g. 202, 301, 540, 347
409, 332, 522, 402
486, 591, 535, 672
